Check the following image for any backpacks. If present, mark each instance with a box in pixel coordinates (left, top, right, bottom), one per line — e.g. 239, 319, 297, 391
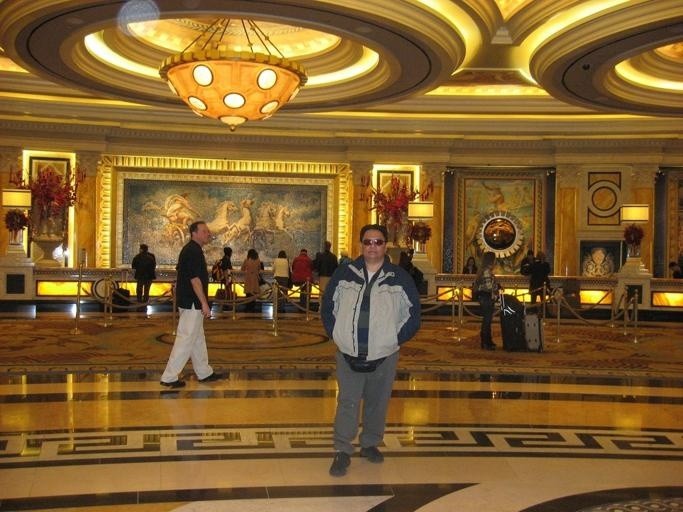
211, 259, 225, 282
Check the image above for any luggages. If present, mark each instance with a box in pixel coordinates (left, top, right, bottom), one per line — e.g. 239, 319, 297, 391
497, 288, 523, 351
523, 312, 545, 352
112, 267, 131, 310
215, 283, 227, 299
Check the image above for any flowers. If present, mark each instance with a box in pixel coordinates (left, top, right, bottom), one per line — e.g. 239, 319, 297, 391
8, 159, 87, 223
368, 168, 420, 227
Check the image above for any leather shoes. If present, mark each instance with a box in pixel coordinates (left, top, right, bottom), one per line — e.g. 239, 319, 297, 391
359, 445, 387, 465
199, 372, 230, 381
158, 379, 186, 388
329, 452, 353, 476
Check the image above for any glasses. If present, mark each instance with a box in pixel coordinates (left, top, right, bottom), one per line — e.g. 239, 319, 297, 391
361, 239, 386, 246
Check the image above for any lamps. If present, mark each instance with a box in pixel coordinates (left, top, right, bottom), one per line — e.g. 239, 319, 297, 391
407, 200, 434, 253
159, 17, 309, 131
619, 203, 649, 258
2, 188, 32, 245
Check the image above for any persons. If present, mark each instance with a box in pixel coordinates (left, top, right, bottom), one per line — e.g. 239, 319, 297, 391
292, 241, 353, 313
271, 251, 293, 313
132, 244, 156, 311
159, 221, 222, 388
462, 249, 550, 351
166, 193, 201, 231
669, 262, 683, 279
465, 210, 480, 258
241, 249, 261, 313
482, 181, 504, 210
399, 251, 414, 275
220, 247, 233, 311
320, 224, 422, 477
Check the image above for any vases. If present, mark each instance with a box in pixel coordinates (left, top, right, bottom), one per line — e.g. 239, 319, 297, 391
46, 217, 55, 236
393, 224, 399, 246
